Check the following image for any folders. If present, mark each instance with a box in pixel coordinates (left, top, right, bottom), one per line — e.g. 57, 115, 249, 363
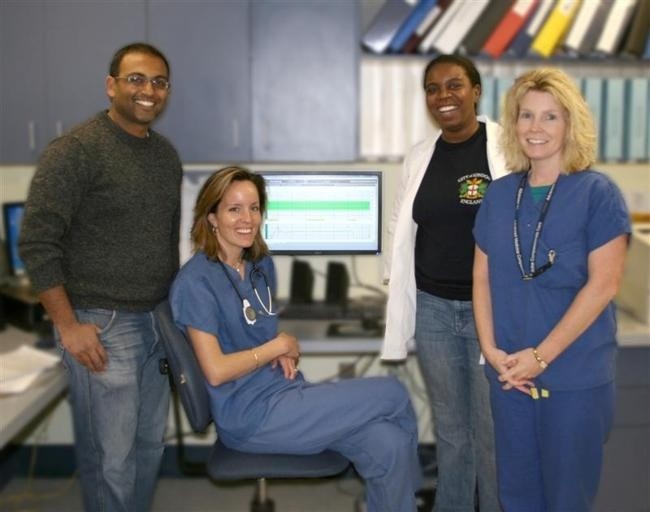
361, 0, 650, 61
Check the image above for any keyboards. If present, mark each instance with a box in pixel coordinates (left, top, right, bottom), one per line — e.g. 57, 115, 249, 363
277, 305, 384, 319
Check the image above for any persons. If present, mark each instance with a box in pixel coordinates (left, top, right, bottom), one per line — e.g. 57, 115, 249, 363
166, 164, 427, 511
468, 64, 634, 512
16, 40, 186, 511
382, 54, 523, 510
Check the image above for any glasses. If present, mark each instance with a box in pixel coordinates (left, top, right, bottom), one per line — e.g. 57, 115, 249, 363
115, 74, 171, 90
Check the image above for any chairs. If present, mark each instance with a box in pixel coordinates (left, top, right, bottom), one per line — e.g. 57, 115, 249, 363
153, 297, 352, 510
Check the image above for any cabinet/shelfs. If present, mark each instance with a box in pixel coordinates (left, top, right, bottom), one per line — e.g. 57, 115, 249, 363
359, 56, 650, 230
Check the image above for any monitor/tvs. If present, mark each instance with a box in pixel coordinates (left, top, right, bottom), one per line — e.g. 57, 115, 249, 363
254, 170, 383, 256
3, 202, 27, 275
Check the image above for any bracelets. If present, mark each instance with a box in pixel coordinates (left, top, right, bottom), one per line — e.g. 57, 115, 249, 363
251, 346, 264, 370
532, 348, 549, 369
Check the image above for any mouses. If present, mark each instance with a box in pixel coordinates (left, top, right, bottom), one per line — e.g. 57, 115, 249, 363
361, 311, 378, 330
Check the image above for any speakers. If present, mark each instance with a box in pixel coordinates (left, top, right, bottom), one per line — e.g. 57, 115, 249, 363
325, 262, 349, 302
289, 261, 314, 303
